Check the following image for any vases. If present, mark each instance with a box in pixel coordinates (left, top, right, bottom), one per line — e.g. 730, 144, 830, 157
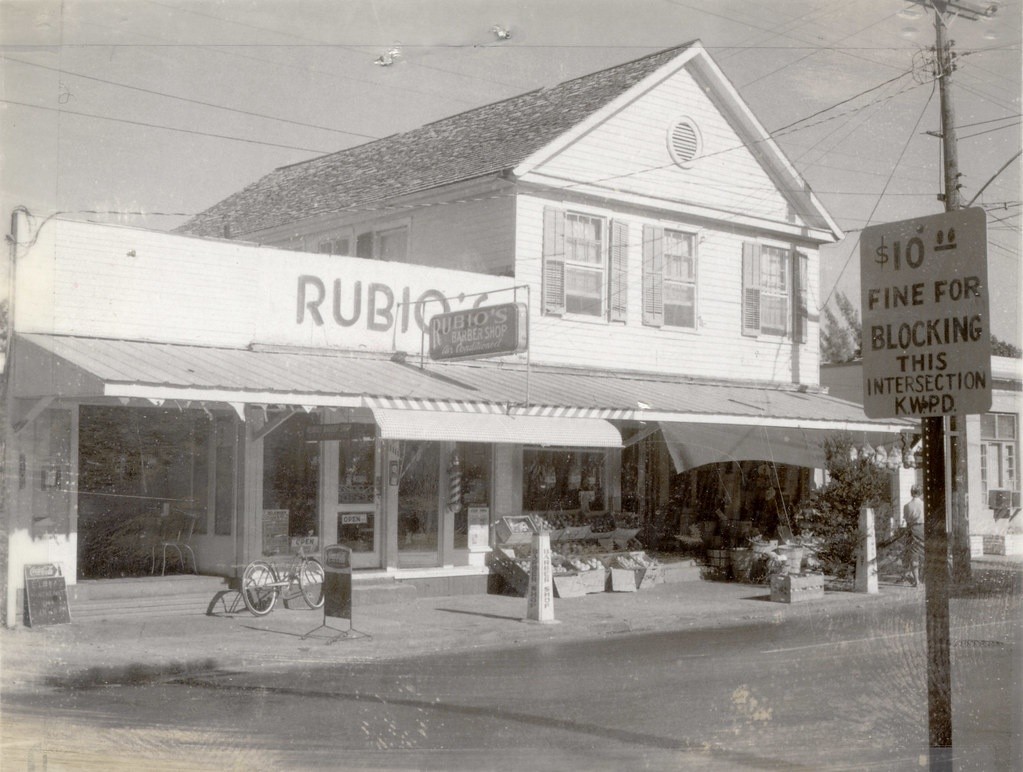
707, 538, 815, 582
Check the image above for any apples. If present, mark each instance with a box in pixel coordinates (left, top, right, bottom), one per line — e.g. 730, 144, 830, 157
534, 513, 615, 532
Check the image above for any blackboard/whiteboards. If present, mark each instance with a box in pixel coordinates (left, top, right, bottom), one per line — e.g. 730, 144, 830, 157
24, 562, 71, 627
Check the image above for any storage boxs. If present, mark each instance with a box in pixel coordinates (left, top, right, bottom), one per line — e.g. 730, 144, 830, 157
494, 510, 660, 598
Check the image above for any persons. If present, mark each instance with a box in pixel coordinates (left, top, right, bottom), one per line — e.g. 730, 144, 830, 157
902, 484, 925, 586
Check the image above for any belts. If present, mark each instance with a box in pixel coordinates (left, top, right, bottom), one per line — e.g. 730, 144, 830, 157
913, 522, 924, 525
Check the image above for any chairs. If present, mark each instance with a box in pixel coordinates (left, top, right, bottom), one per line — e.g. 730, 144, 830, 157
151, 519, 198, 577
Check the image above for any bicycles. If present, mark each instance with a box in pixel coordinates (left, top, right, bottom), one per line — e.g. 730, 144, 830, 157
239, 529, 326, 615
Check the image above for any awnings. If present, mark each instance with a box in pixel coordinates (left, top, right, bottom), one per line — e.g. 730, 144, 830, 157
12, 333, 923, 434
